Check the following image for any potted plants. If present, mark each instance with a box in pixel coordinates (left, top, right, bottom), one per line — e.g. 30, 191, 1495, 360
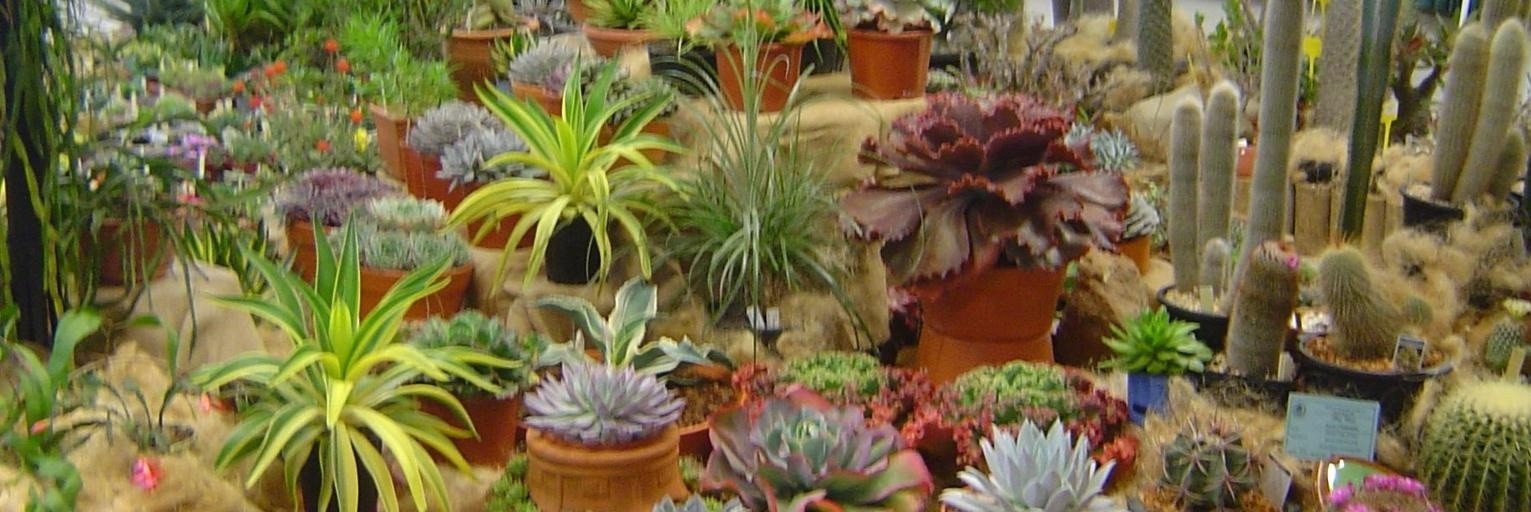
50, 5, 1526, 509
